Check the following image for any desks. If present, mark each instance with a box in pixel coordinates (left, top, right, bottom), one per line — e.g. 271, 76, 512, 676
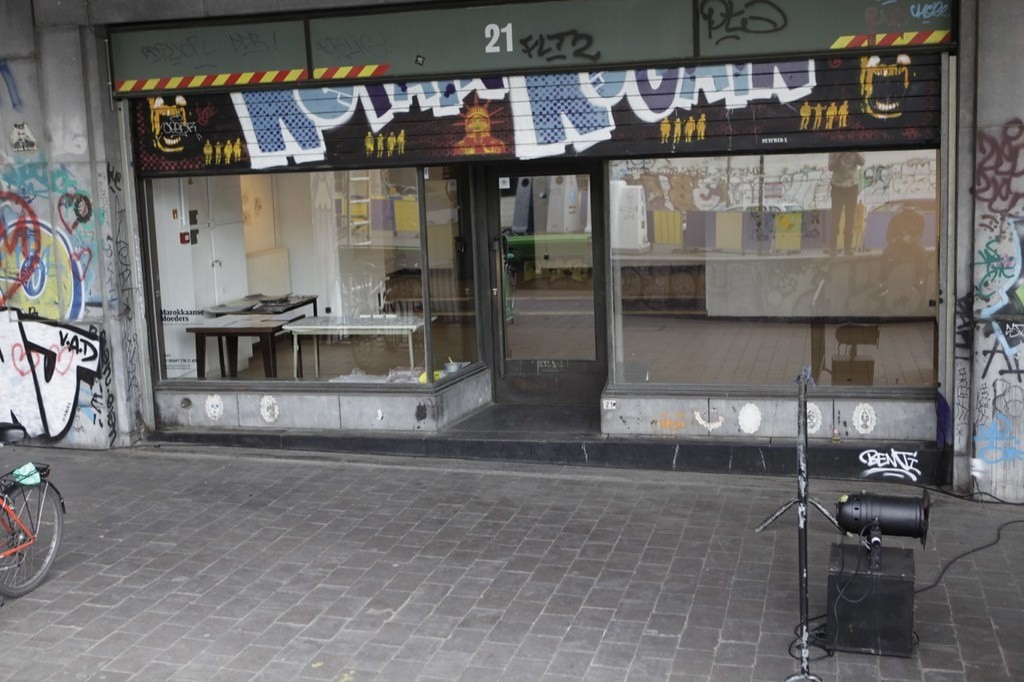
215, 295, 318, 377
283, 316, 438, 380
187, 312, 307, 381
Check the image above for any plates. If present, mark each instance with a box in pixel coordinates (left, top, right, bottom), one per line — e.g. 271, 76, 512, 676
260, 295, 289, 303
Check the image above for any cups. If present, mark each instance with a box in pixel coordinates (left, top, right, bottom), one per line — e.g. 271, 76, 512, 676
444, 362, 463, 376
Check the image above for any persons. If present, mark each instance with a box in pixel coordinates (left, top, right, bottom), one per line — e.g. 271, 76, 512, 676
824, 152, 865, 256
882, 202, 927, 292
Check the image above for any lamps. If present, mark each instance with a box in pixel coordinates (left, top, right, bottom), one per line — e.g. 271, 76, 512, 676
836, 489, 929, 550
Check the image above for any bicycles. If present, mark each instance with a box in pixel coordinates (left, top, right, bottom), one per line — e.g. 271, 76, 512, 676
0, 461, 67, 600
